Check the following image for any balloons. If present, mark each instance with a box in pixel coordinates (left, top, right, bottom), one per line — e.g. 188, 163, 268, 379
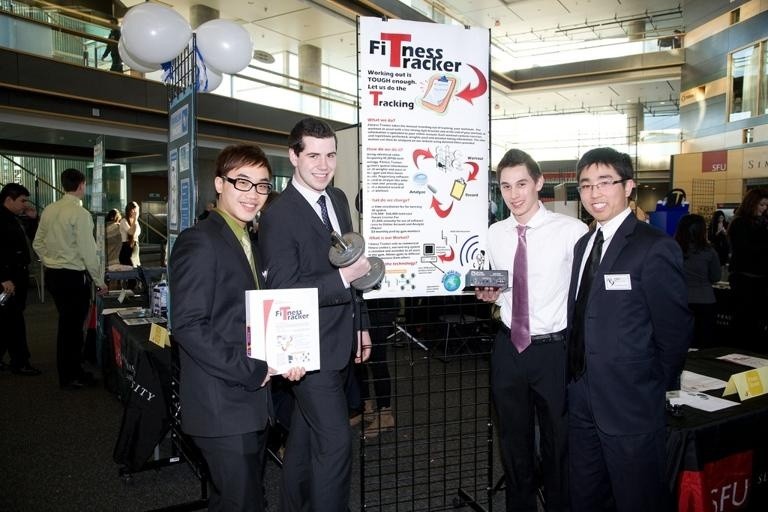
122, 3, 192, 64
118, 36, 164, 74
197, 19, 255, 75
176, 38, 222, 94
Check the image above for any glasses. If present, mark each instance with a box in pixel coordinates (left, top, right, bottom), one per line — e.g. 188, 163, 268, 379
577, 178, 625, 194
222, 175, 272, 195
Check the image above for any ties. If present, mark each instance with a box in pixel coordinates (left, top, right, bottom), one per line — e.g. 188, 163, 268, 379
317, 195, 334, 234
240, 232, 252, 268
567, 228, 603, 382
511, 226, 531, 354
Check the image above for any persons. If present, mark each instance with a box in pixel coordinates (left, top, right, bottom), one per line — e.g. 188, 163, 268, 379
562, 147, 692, 511
350, 299, 398, 439
474, 149, 590, 511
628, 183, 768, 352
121, 201, 143, 289
102, 19, 122, 73
32, 168, 108, 389
256, 118, 374, 512
107, 210, 120, 287
261, 191, 281, 212
199, 202, 215, 220
1, 182, 43, 376
168, 143, 306, 512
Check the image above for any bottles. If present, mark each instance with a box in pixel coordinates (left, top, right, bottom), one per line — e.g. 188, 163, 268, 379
0, 288, 13, 306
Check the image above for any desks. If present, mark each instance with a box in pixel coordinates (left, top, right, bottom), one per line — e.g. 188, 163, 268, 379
666, 346, 767, 512
81, 265, 181, 471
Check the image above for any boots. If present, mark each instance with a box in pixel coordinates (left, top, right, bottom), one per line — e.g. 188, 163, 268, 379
368, 406, 395, 440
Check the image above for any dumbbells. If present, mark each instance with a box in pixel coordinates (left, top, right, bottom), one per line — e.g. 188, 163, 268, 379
328, 230, 386, 291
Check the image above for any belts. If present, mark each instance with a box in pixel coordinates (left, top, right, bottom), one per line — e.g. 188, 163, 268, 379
499, 321, 566, 344
